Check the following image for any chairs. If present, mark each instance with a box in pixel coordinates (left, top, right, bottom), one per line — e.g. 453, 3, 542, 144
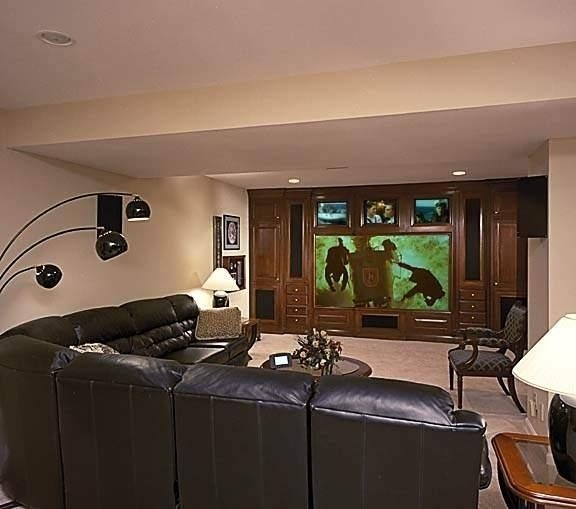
447, 300, 527, 413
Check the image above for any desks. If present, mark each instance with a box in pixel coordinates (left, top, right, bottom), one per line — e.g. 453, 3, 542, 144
491, 432, 576, 509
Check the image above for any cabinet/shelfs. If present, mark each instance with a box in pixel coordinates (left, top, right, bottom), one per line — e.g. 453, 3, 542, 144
223, 255, 246, 293
246, 176, 549, 344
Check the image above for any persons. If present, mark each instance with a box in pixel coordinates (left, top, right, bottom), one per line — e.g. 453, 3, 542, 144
381, 205, 394, 224
432, 202, 449, 223
376, 200, 385, 215
366, 202, 382, 223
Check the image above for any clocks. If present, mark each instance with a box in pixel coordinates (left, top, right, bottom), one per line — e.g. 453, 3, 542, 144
223, 215, 240, 250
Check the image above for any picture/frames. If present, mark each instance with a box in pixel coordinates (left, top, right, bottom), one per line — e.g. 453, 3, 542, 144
314, 199, 352, 229
410, 195, 452, 227
361, 198, 400, 228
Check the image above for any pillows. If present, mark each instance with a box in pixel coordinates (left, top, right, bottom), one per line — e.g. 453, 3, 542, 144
69, 342, 120, 354
194, 307, 242, 341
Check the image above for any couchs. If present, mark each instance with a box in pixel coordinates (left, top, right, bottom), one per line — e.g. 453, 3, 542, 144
0, 294, 488, 509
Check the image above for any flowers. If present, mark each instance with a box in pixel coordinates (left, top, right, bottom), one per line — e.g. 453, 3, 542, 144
292, 327, 343, 377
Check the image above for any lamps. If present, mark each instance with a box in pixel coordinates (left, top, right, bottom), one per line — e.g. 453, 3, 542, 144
201, 268, 240, 308
510, 313, 576, 484
0, 192, 151, 293
